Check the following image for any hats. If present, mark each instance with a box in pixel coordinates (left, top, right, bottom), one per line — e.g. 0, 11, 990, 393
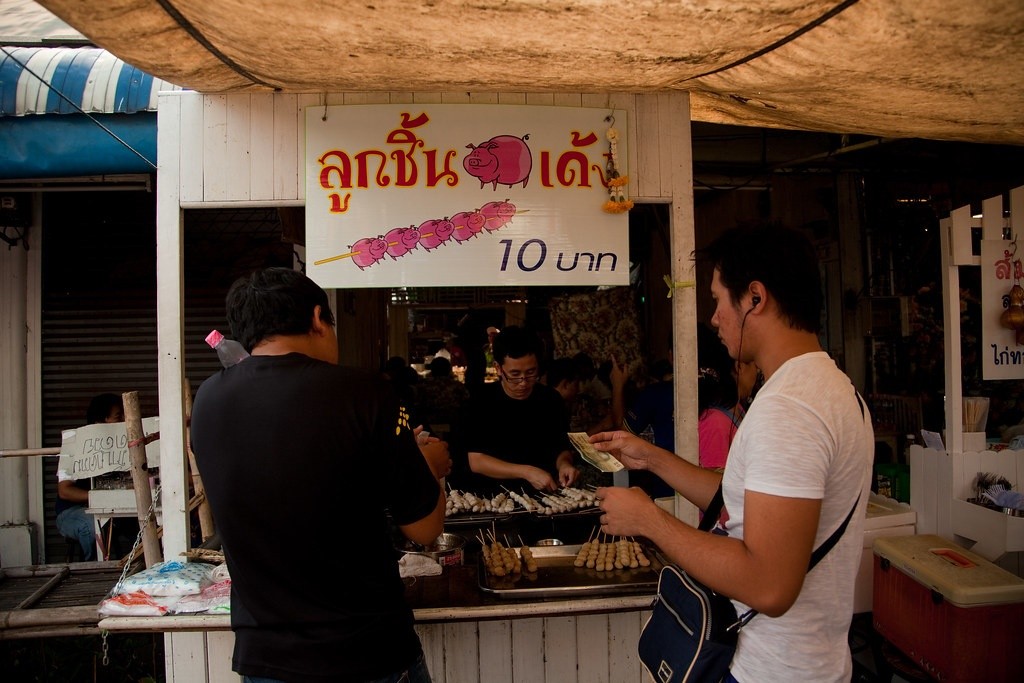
425, 357, 450, 372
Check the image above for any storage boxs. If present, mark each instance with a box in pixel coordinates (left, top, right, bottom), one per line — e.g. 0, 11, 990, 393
870, 534, 1024, 683
86, 485, 157, 508
877, 464, 910, 502
853, 499, 917, 614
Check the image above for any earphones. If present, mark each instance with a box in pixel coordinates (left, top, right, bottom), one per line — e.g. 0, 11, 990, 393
752, 296, 760, 306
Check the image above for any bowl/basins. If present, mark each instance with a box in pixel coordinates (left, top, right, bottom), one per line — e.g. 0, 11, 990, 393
400, 532, 468, 578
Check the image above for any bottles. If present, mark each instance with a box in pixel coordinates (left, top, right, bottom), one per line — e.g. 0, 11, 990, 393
205, 330, 250, 369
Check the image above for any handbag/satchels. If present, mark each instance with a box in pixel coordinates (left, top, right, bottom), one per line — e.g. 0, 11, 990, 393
637, 561, 739, 683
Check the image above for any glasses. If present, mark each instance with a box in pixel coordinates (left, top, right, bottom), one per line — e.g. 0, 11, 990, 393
500, 365, 541, 384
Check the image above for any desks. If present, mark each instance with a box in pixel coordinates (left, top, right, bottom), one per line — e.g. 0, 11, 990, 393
874, 431, 901, 466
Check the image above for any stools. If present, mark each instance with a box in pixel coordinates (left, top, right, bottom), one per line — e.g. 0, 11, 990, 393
63, 526, 123, 563
851, 613, 933, 683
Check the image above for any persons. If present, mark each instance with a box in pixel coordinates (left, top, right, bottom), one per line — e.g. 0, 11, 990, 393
190, 267, 453, 683
460, 325, 581, 494
536, 320, 757, 533
586, 220, 876, 683
53, 393, 125, 562
387, 355, 471, 423
